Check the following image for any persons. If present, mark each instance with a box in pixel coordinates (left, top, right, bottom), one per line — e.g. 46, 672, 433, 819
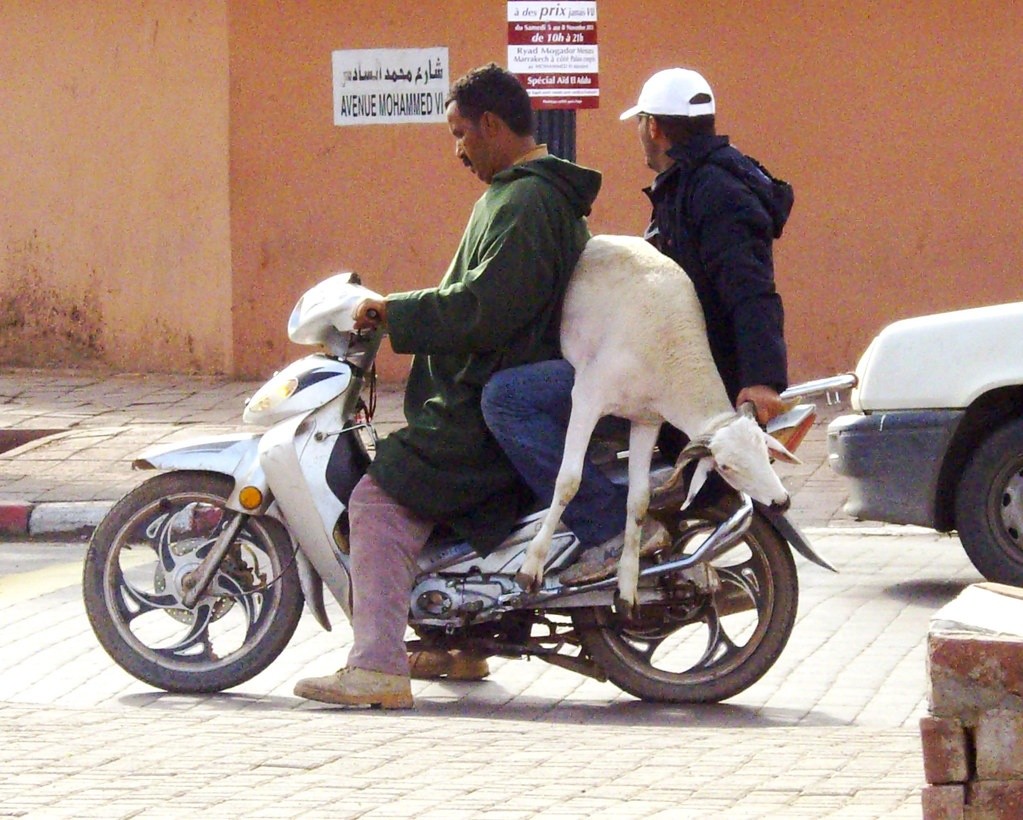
297, 65, 601, 708
481, 67, 796, 585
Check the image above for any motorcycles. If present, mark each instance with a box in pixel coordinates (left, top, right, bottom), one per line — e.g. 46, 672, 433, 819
83, 266, 864, 711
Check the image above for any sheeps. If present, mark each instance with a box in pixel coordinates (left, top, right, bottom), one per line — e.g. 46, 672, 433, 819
511, 233, 801, 625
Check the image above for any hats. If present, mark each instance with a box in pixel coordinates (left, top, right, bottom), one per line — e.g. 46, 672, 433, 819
619, 68, 716, 120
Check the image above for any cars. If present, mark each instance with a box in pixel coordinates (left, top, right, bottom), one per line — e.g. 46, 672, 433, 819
828, 301, 1023, 589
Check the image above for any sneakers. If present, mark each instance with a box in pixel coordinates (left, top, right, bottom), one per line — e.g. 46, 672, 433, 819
408, 651, 489, 680
294, 665, 415, 711
558, 517, 671, 584
